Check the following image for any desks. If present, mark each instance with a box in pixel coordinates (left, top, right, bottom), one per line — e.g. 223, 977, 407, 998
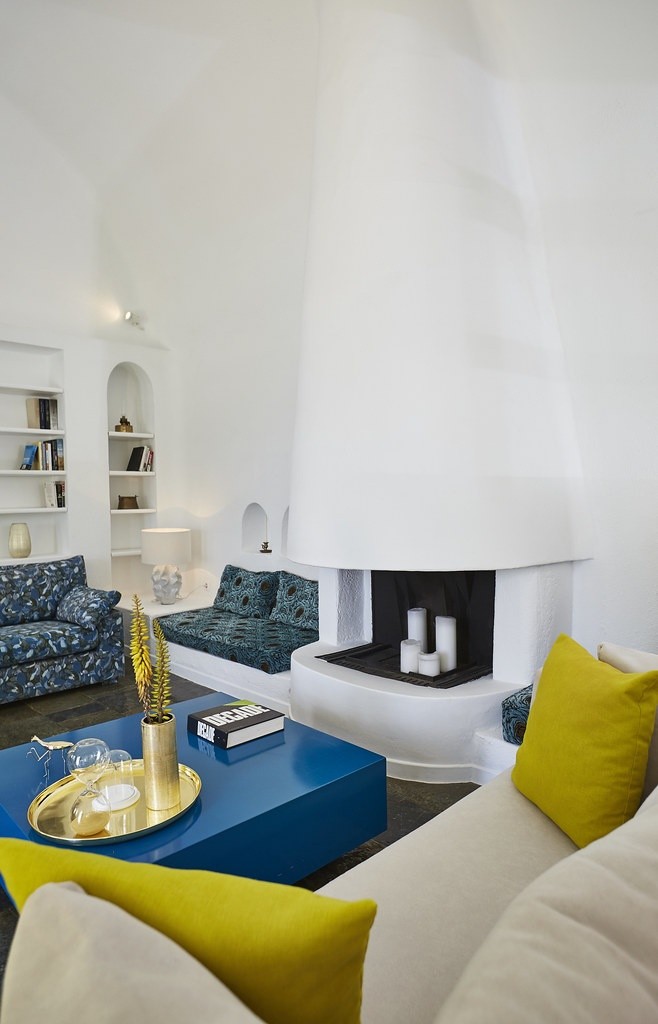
0, 691, 388, 910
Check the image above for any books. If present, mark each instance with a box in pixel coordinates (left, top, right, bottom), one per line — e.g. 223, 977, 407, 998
126, 446, 153, 471
20, 445, 38, 470
31, 439, 64, 470
43, 481, 65, 508
27, 398, 58, 429
187, 698, 285, 750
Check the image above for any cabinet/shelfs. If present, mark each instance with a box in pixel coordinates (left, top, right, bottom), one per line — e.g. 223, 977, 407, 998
108, 362, 158, 585
0, 342, 67, 563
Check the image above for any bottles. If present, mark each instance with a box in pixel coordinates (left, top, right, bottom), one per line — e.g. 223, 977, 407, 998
8, 523, 30, 558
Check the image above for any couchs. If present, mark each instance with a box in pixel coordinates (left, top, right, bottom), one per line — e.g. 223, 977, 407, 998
153, 561, 319, 675
0, 555, 126, 705
0, 642, 658, 1024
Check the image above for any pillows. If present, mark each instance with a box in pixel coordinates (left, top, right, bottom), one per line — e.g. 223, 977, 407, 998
213, 564, 280, 620
55, 584, 121, 631
269, 570, 318, 631
511, 632, 658, 848
0, 836, 378, 1024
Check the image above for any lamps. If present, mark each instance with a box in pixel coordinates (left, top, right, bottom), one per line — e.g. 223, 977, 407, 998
140, 527, 192, 605
